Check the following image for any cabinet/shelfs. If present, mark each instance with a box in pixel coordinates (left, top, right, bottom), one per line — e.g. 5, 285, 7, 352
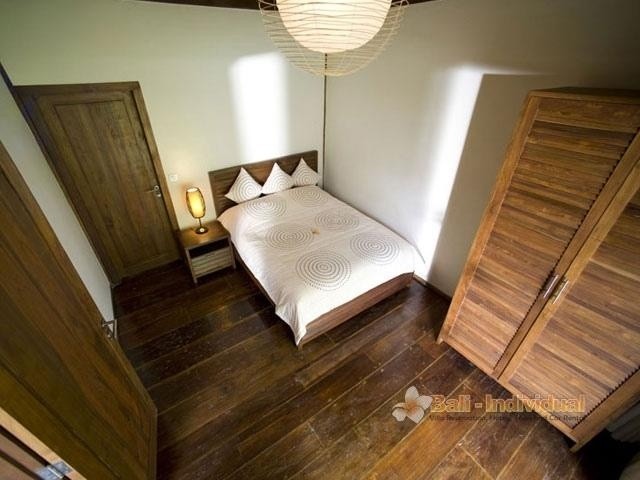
435, 85, 640, 453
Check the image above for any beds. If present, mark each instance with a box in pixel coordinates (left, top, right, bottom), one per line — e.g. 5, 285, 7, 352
208, 151, 416, 351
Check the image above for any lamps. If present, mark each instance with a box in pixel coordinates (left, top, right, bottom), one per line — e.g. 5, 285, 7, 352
258, 1, 409, 78
183, 189, 207, 235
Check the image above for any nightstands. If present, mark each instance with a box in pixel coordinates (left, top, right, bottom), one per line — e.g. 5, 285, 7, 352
174, 220, 237, 284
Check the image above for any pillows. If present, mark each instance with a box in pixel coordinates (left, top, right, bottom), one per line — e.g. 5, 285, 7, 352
225, 158, 323, 204
